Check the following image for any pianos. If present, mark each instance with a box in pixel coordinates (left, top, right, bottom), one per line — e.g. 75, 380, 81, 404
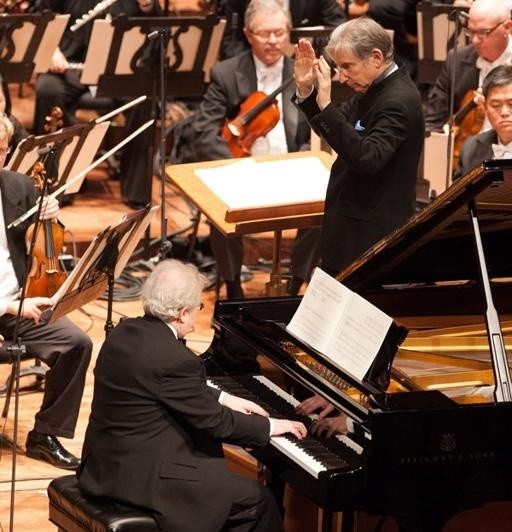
197, 158, 512, 532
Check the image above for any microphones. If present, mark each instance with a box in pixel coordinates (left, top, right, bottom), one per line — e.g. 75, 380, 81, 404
450, 9, 470, 21
148, 28, 168, 42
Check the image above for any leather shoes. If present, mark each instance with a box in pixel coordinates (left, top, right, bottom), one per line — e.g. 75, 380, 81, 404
25, 431, 79, 470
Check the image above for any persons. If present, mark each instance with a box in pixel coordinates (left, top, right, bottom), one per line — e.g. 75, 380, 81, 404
78, 259, 307, 531
320, 1, 436, 91
294, 16, 424, 280
425, 1, 511, 135
191, 1, 314, 160
454, 65, 511, 184
34, 1, 161, 210
1, 113, 93, 469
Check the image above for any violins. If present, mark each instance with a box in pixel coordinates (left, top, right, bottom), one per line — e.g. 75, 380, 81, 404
219, 76, 295, 158
24, 161, 69, 312
447, 90, 485, 181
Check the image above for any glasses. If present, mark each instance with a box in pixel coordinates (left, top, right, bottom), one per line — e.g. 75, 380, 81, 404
334, 60, 366, 76
464, 20, 503, 38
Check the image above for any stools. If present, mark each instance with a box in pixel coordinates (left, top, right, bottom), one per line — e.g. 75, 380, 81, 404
47, 474, 159, 531
